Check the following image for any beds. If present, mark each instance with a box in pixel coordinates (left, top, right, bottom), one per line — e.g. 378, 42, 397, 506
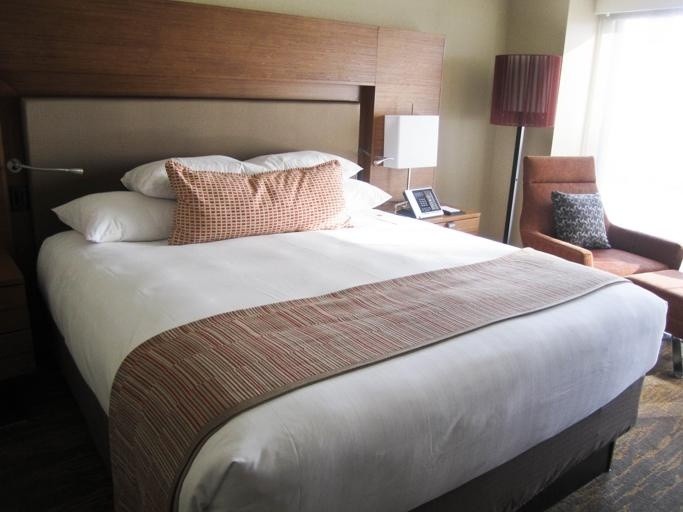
0, 0, 668, 510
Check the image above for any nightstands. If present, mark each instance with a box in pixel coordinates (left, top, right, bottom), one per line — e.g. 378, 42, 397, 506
373, 200, 481, 238
0, 248, 37, 383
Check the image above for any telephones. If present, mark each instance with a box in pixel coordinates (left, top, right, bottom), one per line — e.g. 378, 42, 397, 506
403, 187, 444, 220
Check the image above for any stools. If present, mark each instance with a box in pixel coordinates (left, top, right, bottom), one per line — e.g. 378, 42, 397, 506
623, 269, 683, 379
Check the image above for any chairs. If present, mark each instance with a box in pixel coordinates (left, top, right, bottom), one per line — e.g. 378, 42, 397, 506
517, 155, 682, 280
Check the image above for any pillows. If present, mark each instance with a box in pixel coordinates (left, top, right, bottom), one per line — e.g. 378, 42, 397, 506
547, 189, 610, 249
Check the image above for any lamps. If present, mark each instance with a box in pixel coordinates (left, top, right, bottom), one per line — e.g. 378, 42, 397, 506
383, 101, 439, 218
363, 149, 392, 168
7, 158, 84, 179
489, 53, 562, 244
